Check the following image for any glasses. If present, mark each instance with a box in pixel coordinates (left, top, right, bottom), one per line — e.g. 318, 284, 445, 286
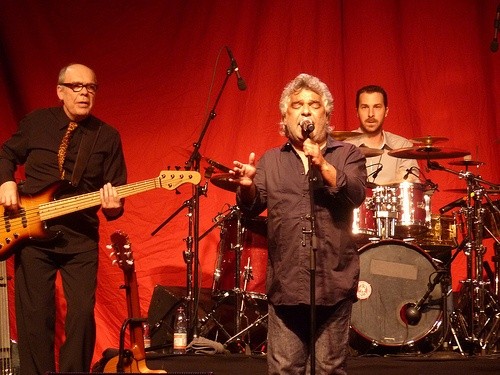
59, 83, 99, 94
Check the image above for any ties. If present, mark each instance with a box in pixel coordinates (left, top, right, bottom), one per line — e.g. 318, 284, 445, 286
57, 121, 79, 181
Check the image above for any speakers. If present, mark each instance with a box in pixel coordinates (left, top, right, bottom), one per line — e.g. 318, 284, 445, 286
147, 283, 269, 354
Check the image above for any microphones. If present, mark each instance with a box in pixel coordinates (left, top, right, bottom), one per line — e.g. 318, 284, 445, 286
439, 197, 467, 214
373, 166, 382, 178
404, 168, 413, 180
406, 307, 421, 322
300, 118, 315, 132
204, 157, 233, 173
226, 47, 247, 91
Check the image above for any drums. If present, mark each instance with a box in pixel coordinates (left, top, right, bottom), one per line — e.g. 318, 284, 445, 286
212, 211, 269, 305
350, 196, 384, 244
413, 214, 458, 254
451, 200, 500, 242
347, 238, 447, 349
383, 182, 432, 239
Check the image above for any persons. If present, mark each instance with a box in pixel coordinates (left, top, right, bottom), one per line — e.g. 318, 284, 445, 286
342, 85, 421, 186
0, 64, 127, 375
228, 74, 368, 375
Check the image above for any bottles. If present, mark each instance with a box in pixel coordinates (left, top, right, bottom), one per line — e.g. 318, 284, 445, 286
173, 307, 188, 354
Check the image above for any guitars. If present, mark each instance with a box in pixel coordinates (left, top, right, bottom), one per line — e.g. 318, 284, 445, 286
105, 228, 166, 374
0, 163, 203, 261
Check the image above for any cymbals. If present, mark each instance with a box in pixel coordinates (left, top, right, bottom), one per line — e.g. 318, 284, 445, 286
444, 187, 500, 195
330, 130, 384, 158
388, 136, 472, 160
447, 161, 486, 166
211, 172, 240, 193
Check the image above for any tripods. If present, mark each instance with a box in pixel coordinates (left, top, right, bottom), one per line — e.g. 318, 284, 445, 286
144, 65, 270, 355
426, 158, 500, 357
